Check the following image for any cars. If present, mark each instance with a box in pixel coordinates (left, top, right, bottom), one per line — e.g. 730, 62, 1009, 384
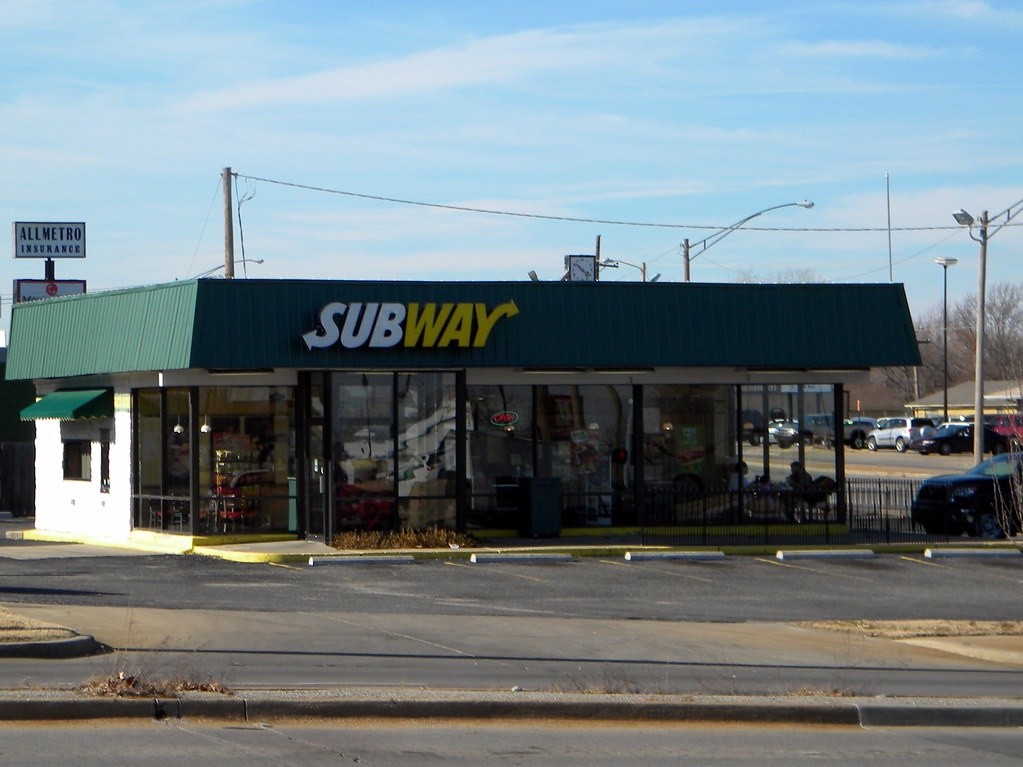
907, 448, 1023, 540
738, 405, 1023, 449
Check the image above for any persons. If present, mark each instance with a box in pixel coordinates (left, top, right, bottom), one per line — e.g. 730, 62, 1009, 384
786, 462, 813, 483
758, 475, 773, 491
729, 462, 750, 489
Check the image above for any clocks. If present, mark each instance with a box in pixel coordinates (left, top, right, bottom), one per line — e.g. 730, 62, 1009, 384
569, 255, 596, 282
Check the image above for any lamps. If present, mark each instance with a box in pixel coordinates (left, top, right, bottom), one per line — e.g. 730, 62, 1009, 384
743, 385, 754, 430
174, 391, 184, 433
588, 422, 600, 430
201, 414, 212, 433
504, 425, 515, 431
662, 423, 674, 431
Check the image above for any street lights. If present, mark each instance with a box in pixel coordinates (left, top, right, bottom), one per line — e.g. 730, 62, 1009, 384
681, 197, 815, 282
931, 255, 958, 422
950, 209, 989, 468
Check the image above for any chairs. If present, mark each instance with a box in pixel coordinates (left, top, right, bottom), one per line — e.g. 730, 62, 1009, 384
636, 467, 828, 524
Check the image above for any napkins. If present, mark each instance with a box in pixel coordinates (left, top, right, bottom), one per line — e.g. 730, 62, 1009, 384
148, 492, 248, 534
